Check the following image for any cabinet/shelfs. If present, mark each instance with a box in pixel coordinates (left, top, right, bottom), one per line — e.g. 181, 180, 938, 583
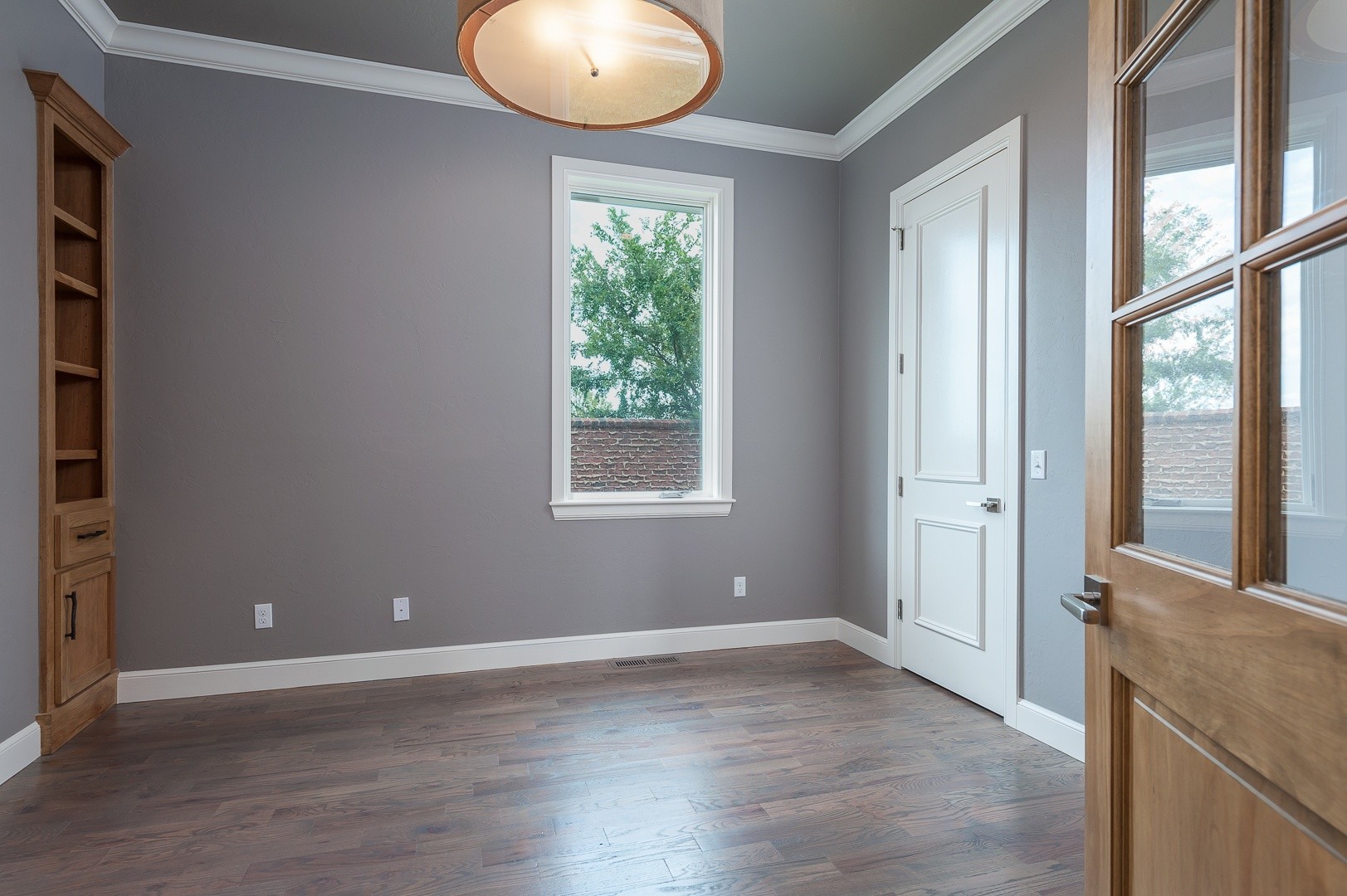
22, 65, 134, 756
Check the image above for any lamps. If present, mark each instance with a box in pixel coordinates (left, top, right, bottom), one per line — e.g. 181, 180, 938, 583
455, 1, 725, 133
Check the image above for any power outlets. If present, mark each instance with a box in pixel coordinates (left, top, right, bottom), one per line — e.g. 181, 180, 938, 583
734, 576, 746, 597
254, 603, 273, 629
